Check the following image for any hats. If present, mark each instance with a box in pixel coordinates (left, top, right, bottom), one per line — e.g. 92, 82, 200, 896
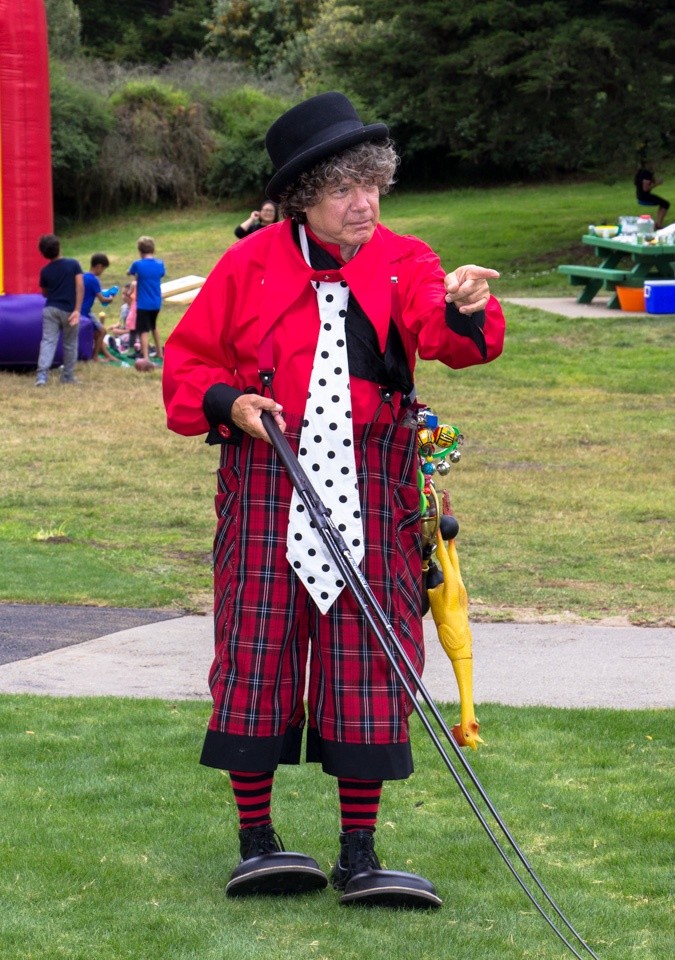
265, 92, 390, 207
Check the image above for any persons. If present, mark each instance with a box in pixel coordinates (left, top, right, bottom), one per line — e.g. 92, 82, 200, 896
159, 88, 510, 914
126, 275, 142, 350
633, 155, 672, 230
34, 235, 86, 388
126, 233, 169, 361
83, 252, 122, 363
234, 202, 279, 239
107, 282, 133, 338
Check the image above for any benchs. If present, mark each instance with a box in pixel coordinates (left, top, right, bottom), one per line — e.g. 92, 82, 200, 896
558, 264, 632, 292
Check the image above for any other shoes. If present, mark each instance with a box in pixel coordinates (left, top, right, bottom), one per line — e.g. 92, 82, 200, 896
225, 822, 327, 897
34, 369, 48, 386
61, 372, 75, 383
328, 830, 443, 910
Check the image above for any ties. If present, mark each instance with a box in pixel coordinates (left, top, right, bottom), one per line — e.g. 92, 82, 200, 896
285, 222, 366, 616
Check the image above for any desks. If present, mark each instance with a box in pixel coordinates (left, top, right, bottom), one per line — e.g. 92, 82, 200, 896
576, 234, 675, 310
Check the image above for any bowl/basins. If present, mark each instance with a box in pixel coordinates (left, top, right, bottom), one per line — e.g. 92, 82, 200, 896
594, 225, 618, 238
616, 284, 646, 310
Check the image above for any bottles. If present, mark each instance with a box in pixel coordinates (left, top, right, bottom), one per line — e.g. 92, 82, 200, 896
636, 214, 655, 237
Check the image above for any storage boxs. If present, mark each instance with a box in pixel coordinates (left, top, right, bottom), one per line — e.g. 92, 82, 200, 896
643, 279, 675, 315
615, 286, 647, 313
